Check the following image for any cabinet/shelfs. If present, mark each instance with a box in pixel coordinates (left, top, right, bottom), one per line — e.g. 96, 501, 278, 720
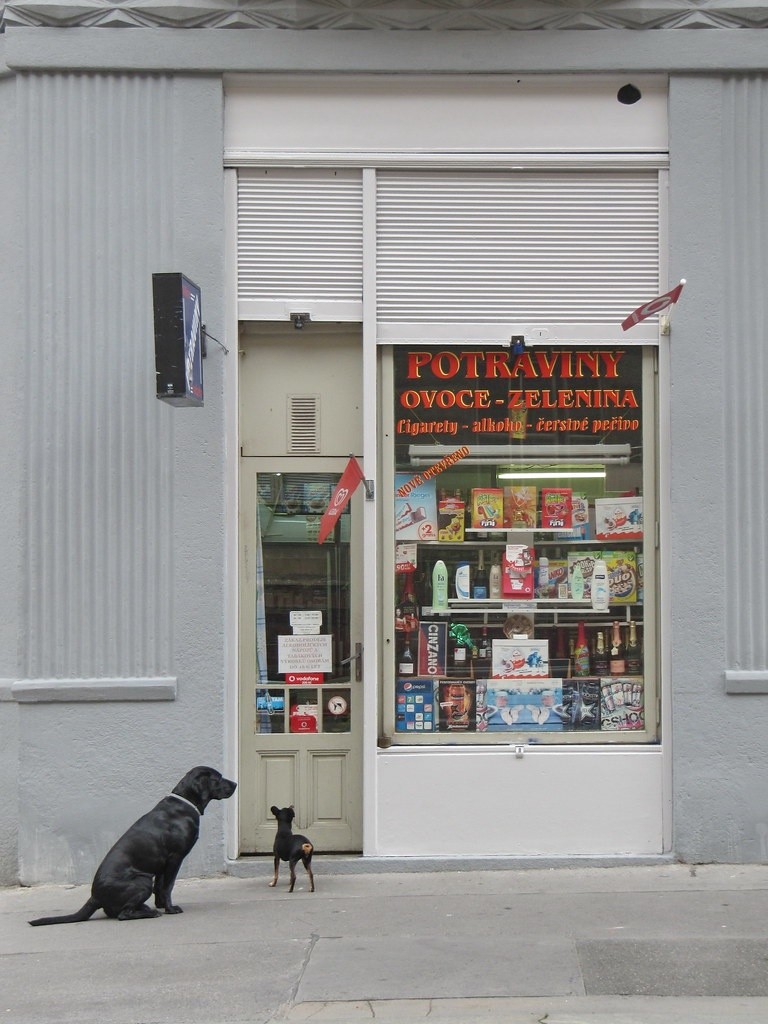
395, 528, 645, 731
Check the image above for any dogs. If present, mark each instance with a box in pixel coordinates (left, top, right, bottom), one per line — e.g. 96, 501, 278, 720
27, 765, 237, 927
268, 805, 315, 893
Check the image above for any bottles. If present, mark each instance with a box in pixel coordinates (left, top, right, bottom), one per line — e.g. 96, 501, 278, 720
479, 626, 490, 658
398, 632, 418, 677
568, 640, 577, 676
594, 632, 608, 676
509, 370, 529, 445
626, 620, 642, 676
574, 621, 590, 677
608, 620, 626, 676
489, 552, 502, 599
451, 630, 467, 677
473, 549, 488, 599
399, 569, 416, 618
591, 636, 597, 675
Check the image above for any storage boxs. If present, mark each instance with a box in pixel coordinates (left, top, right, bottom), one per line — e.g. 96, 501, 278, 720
504, 486, 538, 528
492, 639, 549, 679
439, 502, 465, 542
595, 498, 643, 539
473, 489, 503, 527
547, 560, 568, 598
567, 552, 601, 599
601, 551, 637, 603
541, 488, 572, 528
553, 492, 588, 541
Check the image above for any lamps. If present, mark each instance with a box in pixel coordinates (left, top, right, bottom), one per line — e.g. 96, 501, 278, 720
496, 464, 607, 479
409, 443, 631, 467
